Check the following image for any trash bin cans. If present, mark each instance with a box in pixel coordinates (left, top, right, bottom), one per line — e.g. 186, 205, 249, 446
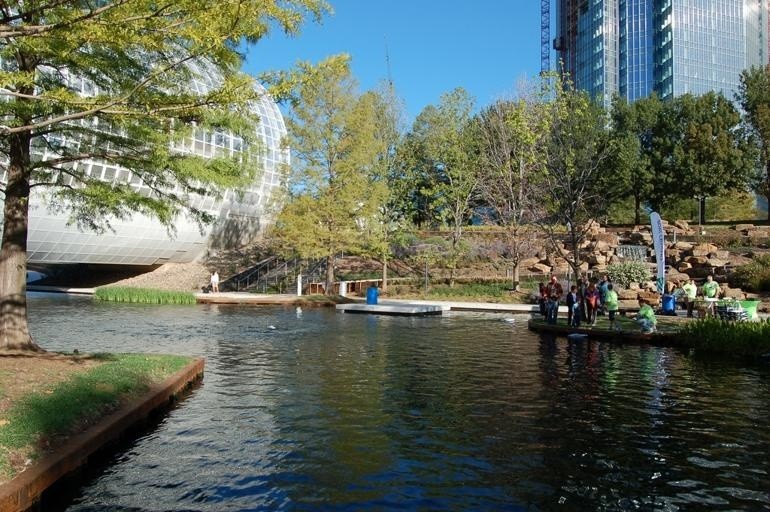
367, 287, 377, 304
662, 294, 675, 314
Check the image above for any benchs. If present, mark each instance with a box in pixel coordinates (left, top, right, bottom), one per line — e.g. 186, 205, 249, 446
617, 307, 664, 316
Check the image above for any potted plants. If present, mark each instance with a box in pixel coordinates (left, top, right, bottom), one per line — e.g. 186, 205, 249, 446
739, 298, 761, 318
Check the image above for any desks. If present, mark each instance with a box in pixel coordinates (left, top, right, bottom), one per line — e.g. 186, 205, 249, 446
692, 297, 741, 318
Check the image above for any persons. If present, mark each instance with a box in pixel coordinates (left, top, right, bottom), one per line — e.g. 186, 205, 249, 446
599, 274, 610, 316
211, 270, 219, 292
605, 284, 622, 331
570, 295, 582, 327
566, 285, 578, 325
629, 299, 657, 335
539, 282, 549, 321
584, 280, 599, 325
702, 275, 721, 319
682, 279, 697, 316
538, 333, 657, 407
546, 276, 563, 324
